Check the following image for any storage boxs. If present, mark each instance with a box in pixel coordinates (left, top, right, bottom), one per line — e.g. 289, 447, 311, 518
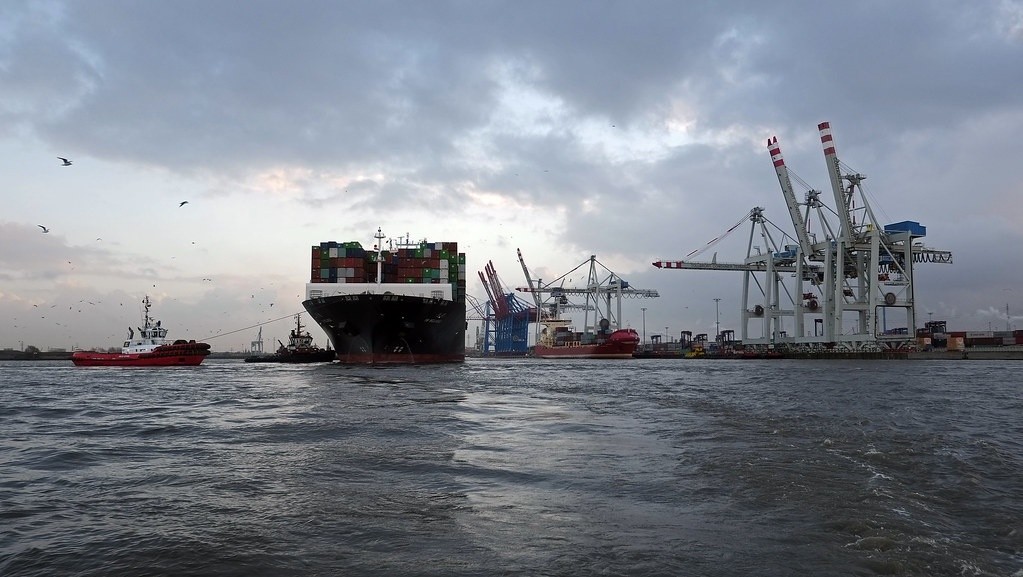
683, 341, 703, 356
916, 321, 1023, 350
311, 241, 466, 304
553, 327, 613, 346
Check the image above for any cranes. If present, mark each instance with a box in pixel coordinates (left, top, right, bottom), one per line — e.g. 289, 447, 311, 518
653, 120, 955, 350
475, 246, 661, 358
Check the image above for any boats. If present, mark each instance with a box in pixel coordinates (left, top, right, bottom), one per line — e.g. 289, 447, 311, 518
72, 295, 212, 367
243, 313, 338, 366
303, 230, 472, 363
529, 320, 640, 359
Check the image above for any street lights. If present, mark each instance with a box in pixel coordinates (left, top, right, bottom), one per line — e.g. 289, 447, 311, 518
640, 307, 649, 349
713, 297, 723, 346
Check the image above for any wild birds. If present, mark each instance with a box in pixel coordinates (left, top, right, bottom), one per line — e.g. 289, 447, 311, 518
57, 156, 73, 166
37, 224, 50, 233
179, 201, 188, 207
